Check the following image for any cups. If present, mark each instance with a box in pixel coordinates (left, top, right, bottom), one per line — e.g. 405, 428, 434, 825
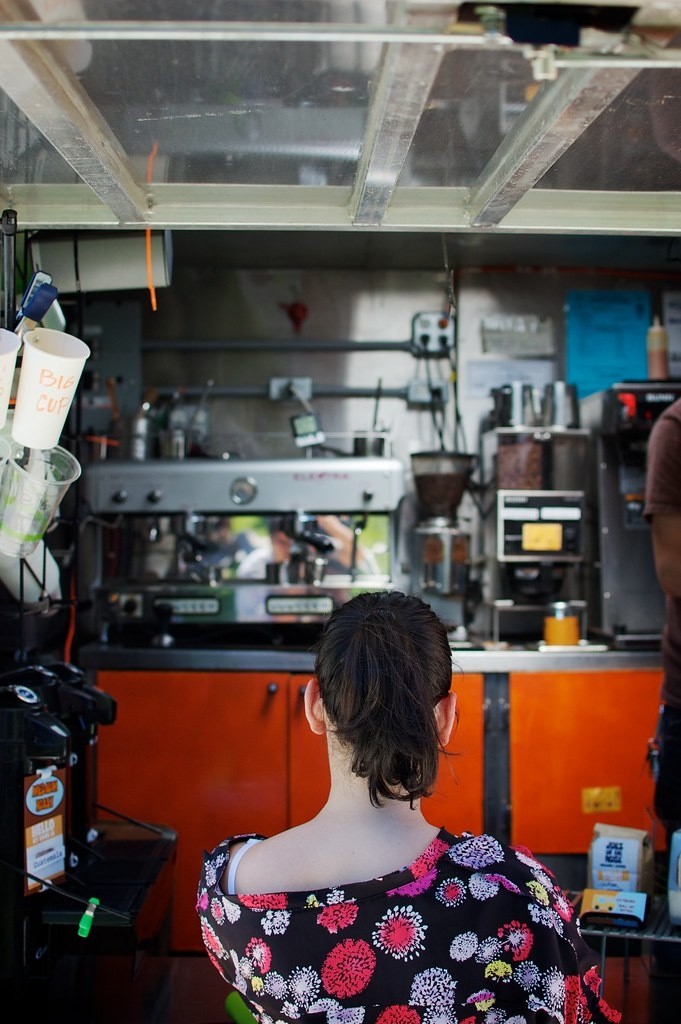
0, 437, 82, 559
353, 429, 384, 456
11, 328, 91, 450
109, 417, 157, 461
0, 328, 22, 430
544, 616, 579, 645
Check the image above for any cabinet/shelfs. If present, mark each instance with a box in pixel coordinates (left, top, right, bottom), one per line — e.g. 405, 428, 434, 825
505, 672, 681, 853
95, 669, 487, 954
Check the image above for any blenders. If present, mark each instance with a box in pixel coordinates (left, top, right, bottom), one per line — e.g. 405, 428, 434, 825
405, 451, 480, 643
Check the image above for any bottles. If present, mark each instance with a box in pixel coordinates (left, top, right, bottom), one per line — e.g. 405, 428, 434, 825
169, 427, 185, 461
647, 316, 670, 380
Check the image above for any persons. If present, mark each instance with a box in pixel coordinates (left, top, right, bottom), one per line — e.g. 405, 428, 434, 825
235, 514, 379, 624
646, 400, 681, 849
196, 591, 621, 1024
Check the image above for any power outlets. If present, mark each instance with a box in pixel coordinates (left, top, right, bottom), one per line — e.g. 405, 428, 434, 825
411, 312, 456, 353
407, 379, 448, 403
267, 377, 313, 401
